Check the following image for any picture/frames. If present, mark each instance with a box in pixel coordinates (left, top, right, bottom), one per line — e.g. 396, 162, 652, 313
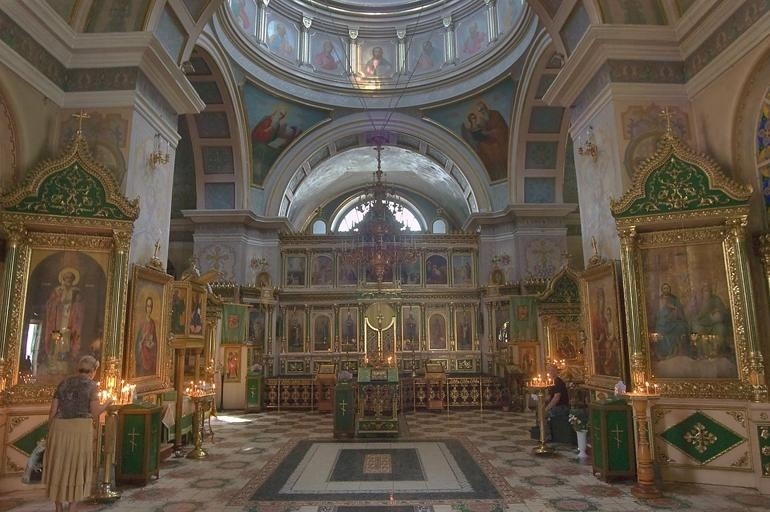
172, 243, 584, 383
617, 223, 754, 401
577, 258, 630, 395
4, 231, 115, 406
124, 262, 175, 395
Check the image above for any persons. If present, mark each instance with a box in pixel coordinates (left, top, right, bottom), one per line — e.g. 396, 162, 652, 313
39, 266, 84, 375
406, 311, 417, 341
653, 282, 689, 361
690, 281, 728, 360
26, 356, 32, 369
135, 296, 158, 376
345, 311, 354, 338
532, 365, 570, 430
600, 308, 619, 377
42, 356, 113, 512
228, 352, 238, 379
460, 311, 471, 345
290, 317, 301, 347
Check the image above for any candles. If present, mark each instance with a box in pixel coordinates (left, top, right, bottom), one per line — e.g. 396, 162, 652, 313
633, 381, 658, 395
154, 134, 170, 154
578, 125, 593, 146
528, 374, 555, 386
94, 376, 137, 406
186, 379, 216, 397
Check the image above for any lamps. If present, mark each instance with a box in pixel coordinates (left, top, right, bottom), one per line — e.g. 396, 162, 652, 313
316, 0, 454, 292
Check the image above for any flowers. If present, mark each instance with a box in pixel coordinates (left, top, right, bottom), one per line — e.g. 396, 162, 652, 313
568, 409, 587, 432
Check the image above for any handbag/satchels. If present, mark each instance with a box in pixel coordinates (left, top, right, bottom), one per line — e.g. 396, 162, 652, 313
21, 443, 45, 484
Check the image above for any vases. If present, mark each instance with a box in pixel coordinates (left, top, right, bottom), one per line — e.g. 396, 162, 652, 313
577, 430, 587, 458
